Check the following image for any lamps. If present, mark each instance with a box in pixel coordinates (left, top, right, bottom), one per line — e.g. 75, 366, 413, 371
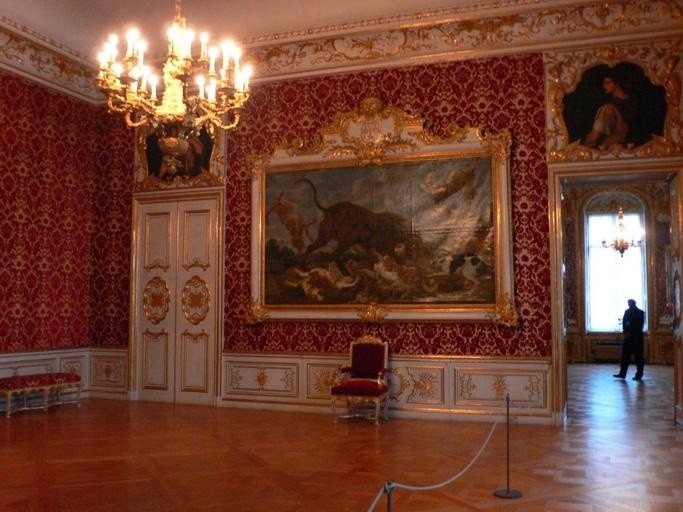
601, 206, 645, 258
95, 1, 255, 167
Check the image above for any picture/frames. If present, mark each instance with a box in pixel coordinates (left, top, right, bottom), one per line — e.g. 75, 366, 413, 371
131, 97, 229, 195
242, 98, 520, 327
541, 34, 683, 166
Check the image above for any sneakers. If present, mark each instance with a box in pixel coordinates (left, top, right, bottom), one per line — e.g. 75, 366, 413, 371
614, 375, 625, 378
632, 377, 641, 380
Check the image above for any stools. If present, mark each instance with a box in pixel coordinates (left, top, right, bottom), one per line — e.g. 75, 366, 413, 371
1, 373, 81, 419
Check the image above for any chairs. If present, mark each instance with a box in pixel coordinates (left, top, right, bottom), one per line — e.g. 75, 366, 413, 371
331, 334, 390, 425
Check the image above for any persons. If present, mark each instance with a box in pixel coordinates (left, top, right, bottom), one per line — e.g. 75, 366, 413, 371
583, 69, 644, 149
612, 296, 646, 383
156, 131, 212, 180
